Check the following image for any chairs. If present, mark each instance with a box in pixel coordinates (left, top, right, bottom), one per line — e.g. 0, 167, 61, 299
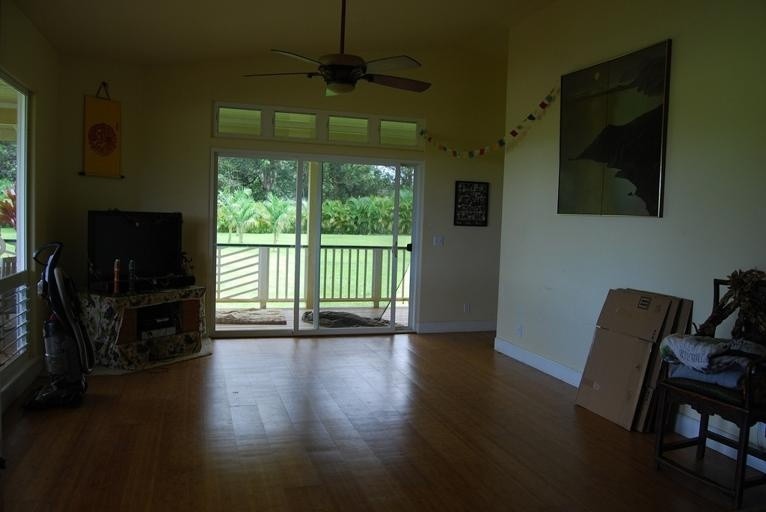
654, 279, 766, 512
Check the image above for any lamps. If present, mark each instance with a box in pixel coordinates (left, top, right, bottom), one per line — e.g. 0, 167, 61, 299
324, 74, 356, 93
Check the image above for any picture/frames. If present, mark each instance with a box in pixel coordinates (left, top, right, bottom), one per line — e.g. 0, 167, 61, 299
454, 180, 488, 226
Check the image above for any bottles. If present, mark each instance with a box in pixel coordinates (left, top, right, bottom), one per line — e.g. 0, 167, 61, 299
128, 260, 136, 280
114, 259, 120, 282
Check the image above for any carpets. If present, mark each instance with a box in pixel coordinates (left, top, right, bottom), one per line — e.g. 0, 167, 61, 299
92, 335, 212, 377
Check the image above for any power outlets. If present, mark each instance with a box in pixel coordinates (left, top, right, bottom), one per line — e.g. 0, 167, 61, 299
433, 236, 443, 245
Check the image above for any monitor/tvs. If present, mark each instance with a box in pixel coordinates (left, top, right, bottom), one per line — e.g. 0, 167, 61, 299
67, 209, 183, 291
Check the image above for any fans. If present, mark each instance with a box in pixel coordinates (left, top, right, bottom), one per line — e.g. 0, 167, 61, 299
244, 0, 430, 94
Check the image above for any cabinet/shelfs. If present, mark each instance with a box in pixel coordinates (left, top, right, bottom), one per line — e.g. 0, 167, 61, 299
72, 285, 208, 368
